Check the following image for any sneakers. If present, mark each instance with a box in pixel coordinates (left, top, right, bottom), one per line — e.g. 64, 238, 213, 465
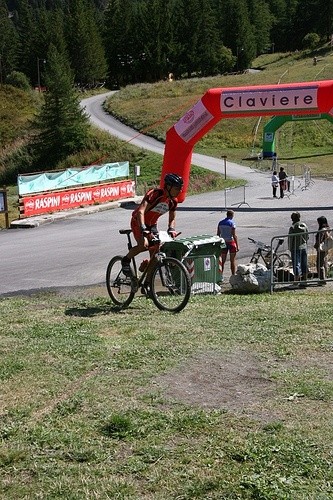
141, 287, 157, 297
121, 256, 131, 277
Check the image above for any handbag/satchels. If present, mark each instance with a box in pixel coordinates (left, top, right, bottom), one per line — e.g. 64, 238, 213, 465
318, 228, 333, 252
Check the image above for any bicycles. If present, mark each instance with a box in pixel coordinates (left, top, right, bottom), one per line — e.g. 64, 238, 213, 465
105, 224, 191, 313
247, 237, 292, 280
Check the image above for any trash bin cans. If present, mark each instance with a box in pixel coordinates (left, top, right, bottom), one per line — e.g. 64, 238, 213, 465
160, 234, 226, 293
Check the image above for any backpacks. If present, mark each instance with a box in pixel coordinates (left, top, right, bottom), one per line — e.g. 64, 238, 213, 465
291, 223, 307, 248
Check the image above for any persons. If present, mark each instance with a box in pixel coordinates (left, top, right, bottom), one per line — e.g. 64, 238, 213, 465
278, 167, 290, 198
217, 210, 240, 282
121, 172, 185, 296
287, 212, 310, 287
313, 215, 333, 285
271, 171, 279, 197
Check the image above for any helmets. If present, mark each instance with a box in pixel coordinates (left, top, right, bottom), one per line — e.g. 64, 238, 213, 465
165, 173, 183, 186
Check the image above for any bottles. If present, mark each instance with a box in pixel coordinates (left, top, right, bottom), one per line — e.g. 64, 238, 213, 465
139, 259, 148, 271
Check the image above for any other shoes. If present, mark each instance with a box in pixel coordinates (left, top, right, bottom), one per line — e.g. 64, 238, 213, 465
318, 282, 326, 285
288, 284, 299, 289
300, 283, 307, 286
222, 279, 224, 281
